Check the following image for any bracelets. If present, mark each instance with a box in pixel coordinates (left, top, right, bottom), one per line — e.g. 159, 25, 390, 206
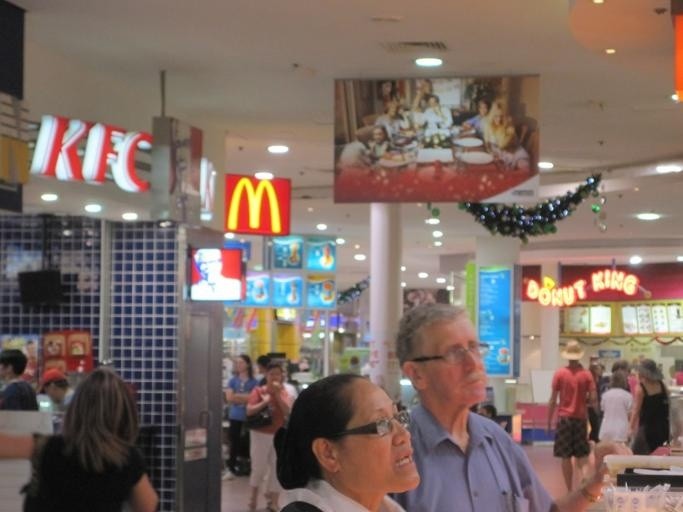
579, 477, 603, 504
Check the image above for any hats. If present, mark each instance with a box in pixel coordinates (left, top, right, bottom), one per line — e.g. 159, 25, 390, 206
36, 369, 68, 393
561, 341, 585, 361
639, 359, 662, 381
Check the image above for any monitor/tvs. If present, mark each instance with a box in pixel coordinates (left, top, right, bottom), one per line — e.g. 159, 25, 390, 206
18, 270, 63, 306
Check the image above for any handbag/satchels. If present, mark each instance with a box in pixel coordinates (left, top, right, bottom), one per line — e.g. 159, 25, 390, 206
247, 408, 271, 428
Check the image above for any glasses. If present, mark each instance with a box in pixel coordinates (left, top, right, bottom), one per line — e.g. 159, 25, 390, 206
412, 344, 490, 365
330, 411, 411, 437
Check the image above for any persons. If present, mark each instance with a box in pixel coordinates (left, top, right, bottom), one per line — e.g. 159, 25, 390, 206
244, 364, 298, 511
270, 373, 423, 510
387, 302, 636, 512
186, 247, 241, 300
338, 79, 531, 177
225, 354, 259, 476
21, 366, 158, 511
621, 358, 670, 455
33, 369, 74, 410
544, 339, 598, 496
582, 355, 633, 444
0, 349, 37, 411
